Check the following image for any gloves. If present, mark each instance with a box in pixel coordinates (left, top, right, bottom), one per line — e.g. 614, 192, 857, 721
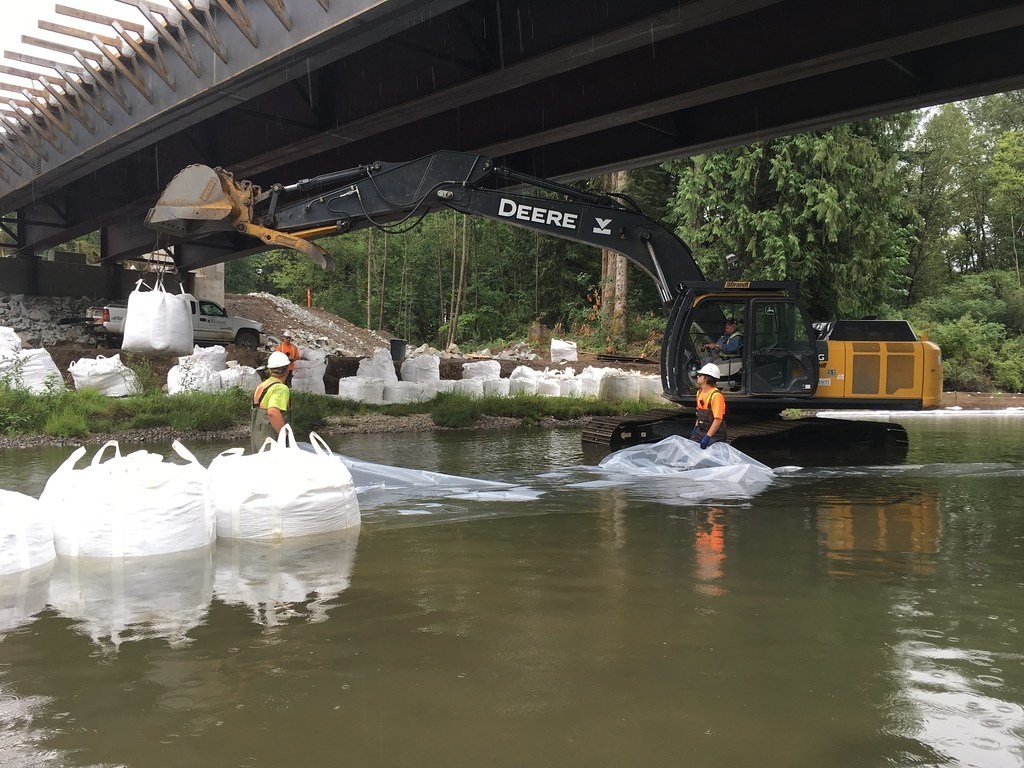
691, 426, 698, 435
285, 351, 290, 356
700, 435, 711, 450
289, 358, 294, 362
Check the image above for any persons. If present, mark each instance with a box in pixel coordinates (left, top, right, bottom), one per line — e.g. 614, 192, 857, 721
275, 332, 300, 388
690, 363, 726, 450
689, 506, 729, 602
251, 351, 291, 453
700, 318, 744, 370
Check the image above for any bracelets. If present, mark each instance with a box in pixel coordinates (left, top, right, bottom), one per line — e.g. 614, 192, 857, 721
716, 344, 719, 348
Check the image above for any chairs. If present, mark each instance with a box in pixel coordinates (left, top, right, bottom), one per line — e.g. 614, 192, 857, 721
714, 334, 757, 376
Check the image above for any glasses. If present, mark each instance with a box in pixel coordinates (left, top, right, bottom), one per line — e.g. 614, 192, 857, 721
285, 337, 291, 339
698, 374, 705, 377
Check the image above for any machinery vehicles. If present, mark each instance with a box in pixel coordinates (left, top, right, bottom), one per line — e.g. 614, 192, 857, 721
144, 148, 945, 467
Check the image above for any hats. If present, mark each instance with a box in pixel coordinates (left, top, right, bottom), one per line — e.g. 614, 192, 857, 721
726, 318, 738, 326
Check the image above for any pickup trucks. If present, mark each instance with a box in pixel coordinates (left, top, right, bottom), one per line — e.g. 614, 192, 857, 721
86, 298, 266, 351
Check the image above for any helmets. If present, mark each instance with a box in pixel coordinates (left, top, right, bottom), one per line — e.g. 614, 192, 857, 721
284, 332, 292, 336
267, 351, 291, 368
697, 363, 720, 379
